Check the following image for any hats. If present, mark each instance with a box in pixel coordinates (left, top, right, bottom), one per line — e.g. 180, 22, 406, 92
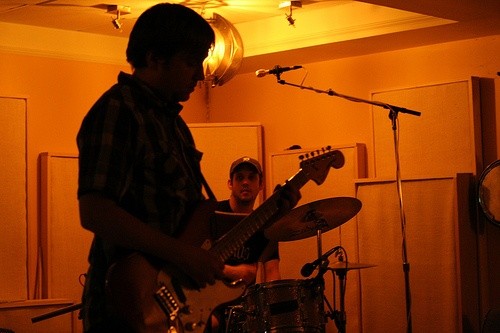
229, 156, 263, 175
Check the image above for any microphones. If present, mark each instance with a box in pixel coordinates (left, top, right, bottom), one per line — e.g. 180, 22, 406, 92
256, 66, 303, 78
301, 247, 339, 277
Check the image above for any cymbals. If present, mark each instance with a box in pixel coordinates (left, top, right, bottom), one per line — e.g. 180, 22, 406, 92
311, 261, 375, 270
263, 197, 362, 244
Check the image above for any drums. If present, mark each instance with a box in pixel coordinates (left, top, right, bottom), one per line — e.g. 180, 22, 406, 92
226, 306, 244, 333
243, 278, 328, 333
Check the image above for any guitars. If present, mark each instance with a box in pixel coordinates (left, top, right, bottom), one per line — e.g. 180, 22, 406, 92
105, 144, 345, 333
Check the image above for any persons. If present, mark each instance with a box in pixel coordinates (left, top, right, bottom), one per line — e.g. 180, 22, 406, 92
77, 2, 302, 333
192, 156, 283, 333
